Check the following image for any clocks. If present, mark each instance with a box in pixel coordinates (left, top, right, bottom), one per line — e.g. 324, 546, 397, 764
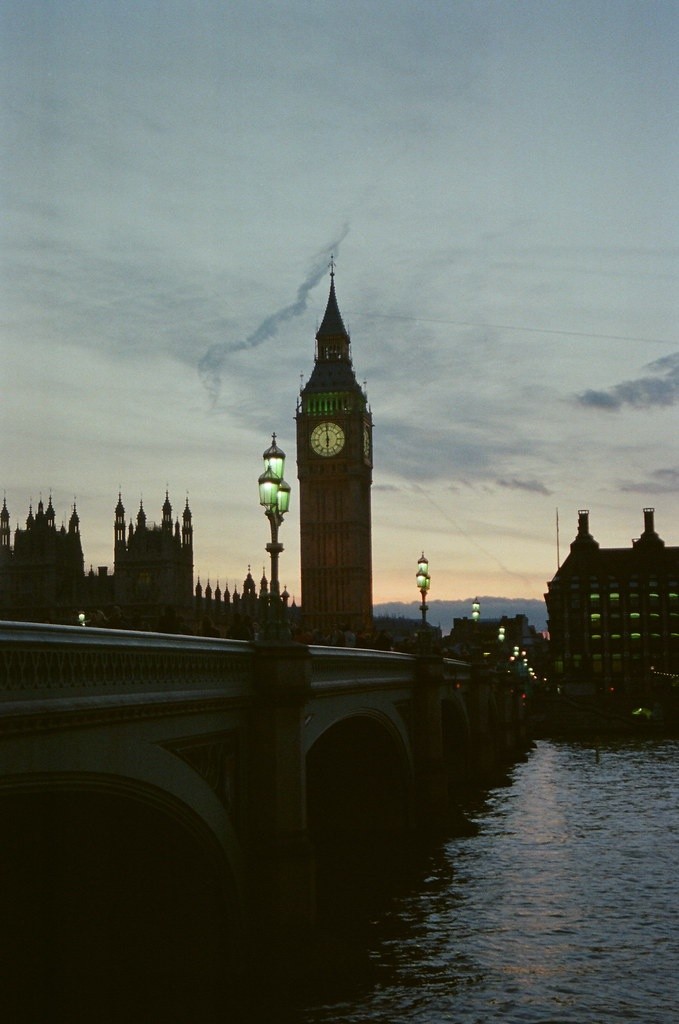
309, 420, 346, 457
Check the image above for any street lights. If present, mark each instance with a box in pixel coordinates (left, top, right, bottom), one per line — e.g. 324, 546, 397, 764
416, 550, 432, 655
257, 431, 292, 641
